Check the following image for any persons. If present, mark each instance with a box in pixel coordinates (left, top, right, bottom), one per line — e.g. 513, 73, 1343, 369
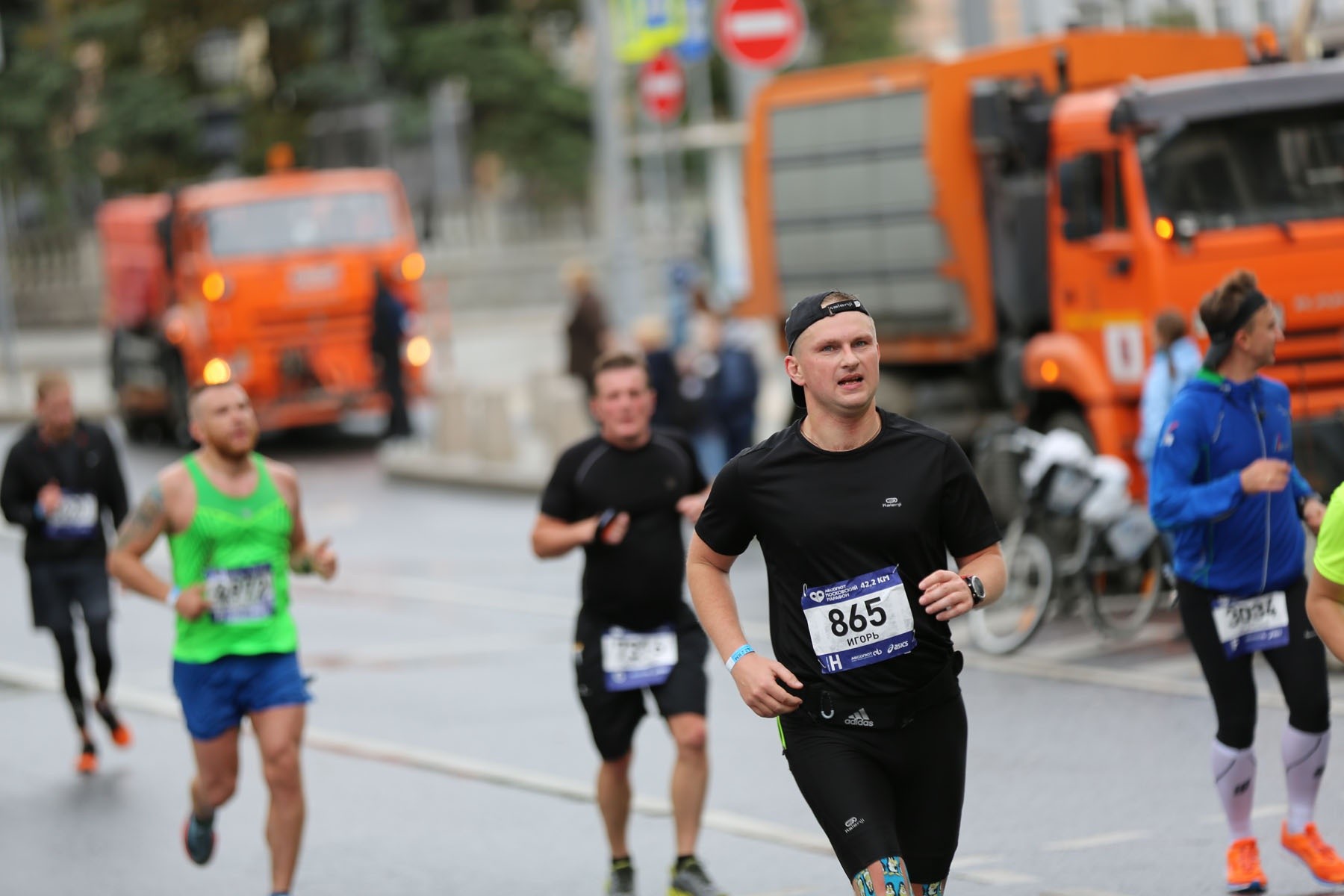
560, 255, 612, 403
1305, 481, 1344, 665
686, 290, 1007, 896
0, 374, 129, 775
109, 381, 337, 896
371, 264, 416, 439
640, 261, 757, 473
1146, 270, 1344, 896
532, 352, 714, 895
1133, 308, 1203, 474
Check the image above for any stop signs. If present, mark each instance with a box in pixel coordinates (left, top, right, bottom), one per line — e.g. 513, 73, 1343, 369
717, 0, 805, 61
637, 53, 685, 127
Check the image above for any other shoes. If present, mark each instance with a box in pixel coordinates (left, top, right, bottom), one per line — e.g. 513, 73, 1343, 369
607, 866, 634, 896
183, 808, 214, 865
669, 860, 724, 895
95, 699, 130, 744
78, 741, 95, 772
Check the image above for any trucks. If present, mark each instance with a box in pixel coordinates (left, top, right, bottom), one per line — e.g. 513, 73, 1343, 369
91, 140, 426, 458
746, 23, 1344, 591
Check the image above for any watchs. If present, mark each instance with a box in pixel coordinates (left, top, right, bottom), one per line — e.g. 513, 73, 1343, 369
960, 575, 986, 607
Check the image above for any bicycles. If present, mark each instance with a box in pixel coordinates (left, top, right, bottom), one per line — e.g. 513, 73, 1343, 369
963, 422, 1167, 656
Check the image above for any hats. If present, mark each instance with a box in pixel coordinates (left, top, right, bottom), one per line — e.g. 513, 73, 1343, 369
784, 292, 870, 408
1201, 291, 1266, 371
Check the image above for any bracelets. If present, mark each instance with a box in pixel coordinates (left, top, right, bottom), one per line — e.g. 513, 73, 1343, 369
726, 644, 754, 673
303, 549, 312, 574
165, 587, 184, 609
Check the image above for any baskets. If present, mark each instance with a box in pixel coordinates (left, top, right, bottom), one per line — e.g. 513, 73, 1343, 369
1003, 448, 1101, 521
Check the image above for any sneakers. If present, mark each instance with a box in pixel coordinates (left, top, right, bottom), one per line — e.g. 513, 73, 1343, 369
1278, 820, 1344, 886
1227, 837, 1267, 894
